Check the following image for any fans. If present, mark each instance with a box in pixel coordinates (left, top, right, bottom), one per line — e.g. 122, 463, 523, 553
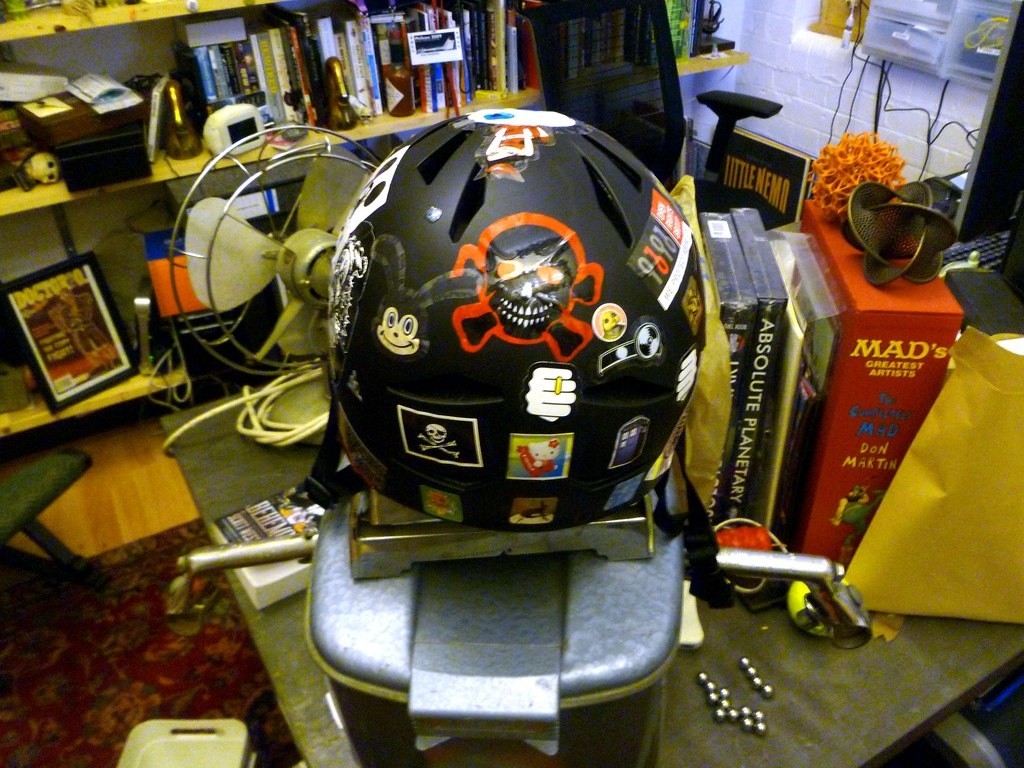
166, 123, 382, 445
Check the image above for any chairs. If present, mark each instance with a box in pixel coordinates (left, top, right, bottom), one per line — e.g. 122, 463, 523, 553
0, 449, 295, 768
517, 0, 789, 229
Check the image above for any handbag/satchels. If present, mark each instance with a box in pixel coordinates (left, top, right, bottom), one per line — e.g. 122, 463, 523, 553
841, 325, 1024, 624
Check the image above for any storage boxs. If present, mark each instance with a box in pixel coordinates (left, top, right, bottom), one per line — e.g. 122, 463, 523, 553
773, 199, 964, 568
19, 85, 152, 192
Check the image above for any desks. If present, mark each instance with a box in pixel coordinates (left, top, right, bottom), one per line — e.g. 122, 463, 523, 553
161, 388, 1024, 768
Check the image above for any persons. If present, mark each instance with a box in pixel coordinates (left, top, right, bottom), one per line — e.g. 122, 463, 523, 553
36, 274, 118, 372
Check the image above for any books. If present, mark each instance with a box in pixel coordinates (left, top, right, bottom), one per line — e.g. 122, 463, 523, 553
174, 0, 518, 126
531, 0, 692, 91
214, 482, 311, 610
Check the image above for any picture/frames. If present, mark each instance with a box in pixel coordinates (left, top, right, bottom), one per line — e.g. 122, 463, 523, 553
0, 250, 139, 415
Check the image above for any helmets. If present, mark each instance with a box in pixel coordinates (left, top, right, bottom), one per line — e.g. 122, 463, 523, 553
328, 111, 707, 532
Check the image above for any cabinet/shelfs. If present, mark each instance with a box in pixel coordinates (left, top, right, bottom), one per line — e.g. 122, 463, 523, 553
0, 0, 749, 450
